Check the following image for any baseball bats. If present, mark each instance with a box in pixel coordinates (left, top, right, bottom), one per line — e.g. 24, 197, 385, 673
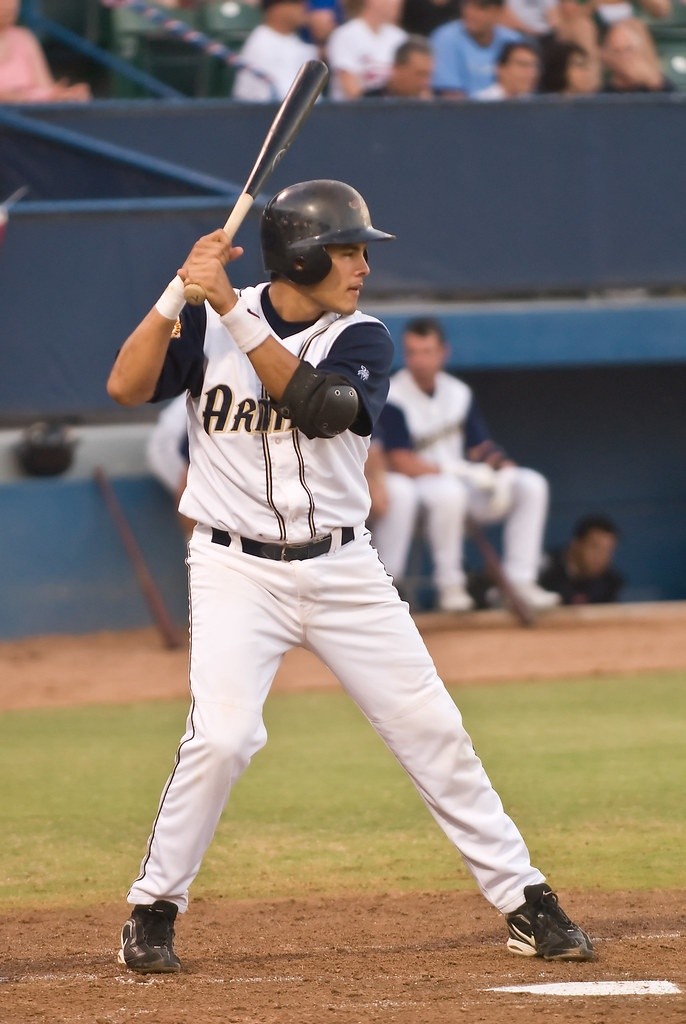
179, 56, 332, 309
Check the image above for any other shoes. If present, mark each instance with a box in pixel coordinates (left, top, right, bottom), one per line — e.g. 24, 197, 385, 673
498, 581, 561, 610
436, 587, 472, 612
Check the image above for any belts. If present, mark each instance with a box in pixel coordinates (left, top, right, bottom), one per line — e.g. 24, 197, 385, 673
211, 527, 354, 562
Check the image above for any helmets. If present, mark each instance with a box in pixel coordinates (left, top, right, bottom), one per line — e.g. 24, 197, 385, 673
261, 179, 395, 286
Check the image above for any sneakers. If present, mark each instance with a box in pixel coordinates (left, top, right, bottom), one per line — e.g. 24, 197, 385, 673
118, 900, 181, 973
504, 885, 590, 961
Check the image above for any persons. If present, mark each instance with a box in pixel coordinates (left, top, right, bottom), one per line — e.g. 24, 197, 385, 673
104, 178, 592, 970
149, 312, 619, 613
0, 0, 672, 119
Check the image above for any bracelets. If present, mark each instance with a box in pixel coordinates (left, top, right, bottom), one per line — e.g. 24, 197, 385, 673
152, 273, 191, 321
219, 298, 270, 354
